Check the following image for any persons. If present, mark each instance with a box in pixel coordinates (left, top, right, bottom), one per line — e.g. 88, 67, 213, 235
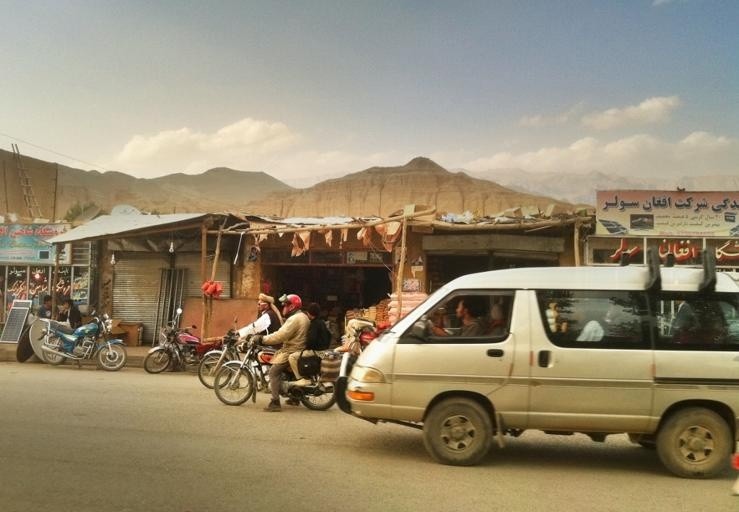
672, 301, 715, 345
249, 293, 311, 411
37, 295, 58, 320
427, 296, 487, 340
228, 292, 284, 391
570, 299, 611, 343
58, 299, 82, 329
285, 302, 332, 406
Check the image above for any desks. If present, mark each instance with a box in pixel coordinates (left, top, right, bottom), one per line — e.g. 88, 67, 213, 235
109, 321, 144, 347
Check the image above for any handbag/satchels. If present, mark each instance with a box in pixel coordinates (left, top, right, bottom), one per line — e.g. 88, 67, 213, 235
298, 355, 322, 377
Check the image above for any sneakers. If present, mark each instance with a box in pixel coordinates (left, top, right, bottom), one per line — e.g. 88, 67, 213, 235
285, 399, 300, 406
263, 399, 282, 411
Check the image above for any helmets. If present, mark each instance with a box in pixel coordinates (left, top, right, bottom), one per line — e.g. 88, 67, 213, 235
279, 293, 302, 317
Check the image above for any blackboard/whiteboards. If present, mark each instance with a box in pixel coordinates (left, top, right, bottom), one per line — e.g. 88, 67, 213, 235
0, 299, 32, 344
38, 291, 47, 305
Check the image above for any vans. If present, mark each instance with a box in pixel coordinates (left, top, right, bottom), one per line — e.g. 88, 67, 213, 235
343, 265, 739, 479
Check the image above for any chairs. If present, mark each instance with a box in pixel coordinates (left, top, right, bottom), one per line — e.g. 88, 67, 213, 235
483, 303, 504, 336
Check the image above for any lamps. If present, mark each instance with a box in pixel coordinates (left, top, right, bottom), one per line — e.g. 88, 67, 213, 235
169, 231, 175, 253
110, 238, 116, 264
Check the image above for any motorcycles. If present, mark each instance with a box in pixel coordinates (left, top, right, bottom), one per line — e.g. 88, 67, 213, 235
143, 307, 224, 373
38, 303, 127, 372
198, 315, 337, 410
